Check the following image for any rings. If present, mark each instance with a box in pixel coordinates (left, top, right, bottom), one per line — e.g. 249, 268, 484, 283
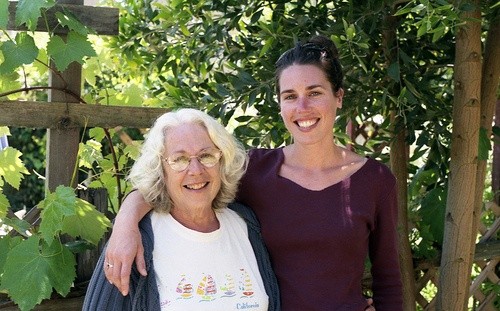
106, 263, 113, 268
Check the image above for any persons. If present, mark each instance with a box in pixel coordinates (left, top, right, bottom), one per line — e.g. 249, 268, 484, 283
78, 106, 285, 311
103, 39, 406, 311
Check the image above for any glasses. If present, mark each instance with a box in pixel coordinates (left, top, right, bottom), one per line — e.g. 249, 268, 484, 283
161, 147, 223, 171
